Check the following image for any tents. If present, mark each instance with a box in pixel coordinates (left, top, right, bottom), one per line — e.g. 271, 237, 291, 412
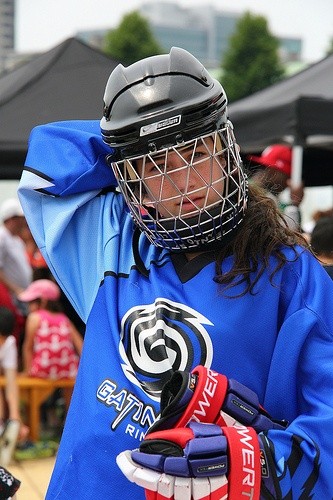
0, 36, 159, 180
227, 53, 333, 142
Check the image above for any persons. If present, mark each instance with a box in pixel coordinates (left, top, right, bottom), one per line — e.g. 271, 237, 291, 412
16, 46, 333, 500
250, 143, 332, 245
1, 204, 82, 500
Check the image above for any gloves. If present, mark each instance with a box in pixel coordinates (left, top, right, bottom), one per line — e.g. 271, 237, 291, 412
115, 364, 283, 500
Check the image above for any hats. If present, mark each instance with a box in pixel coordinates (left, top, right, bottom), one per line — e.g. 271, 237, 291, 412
249, 144, 292, 177
0, 197, 25, 222
16, 279, 60, 302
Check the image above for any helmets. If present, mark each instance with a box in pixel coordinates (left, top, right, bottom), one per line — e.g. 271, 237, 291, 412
98, 47, 248, 254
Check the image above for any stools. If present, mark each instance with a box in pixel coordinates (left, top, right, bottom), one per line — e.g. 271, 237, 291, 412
2, 375, 75, 442
